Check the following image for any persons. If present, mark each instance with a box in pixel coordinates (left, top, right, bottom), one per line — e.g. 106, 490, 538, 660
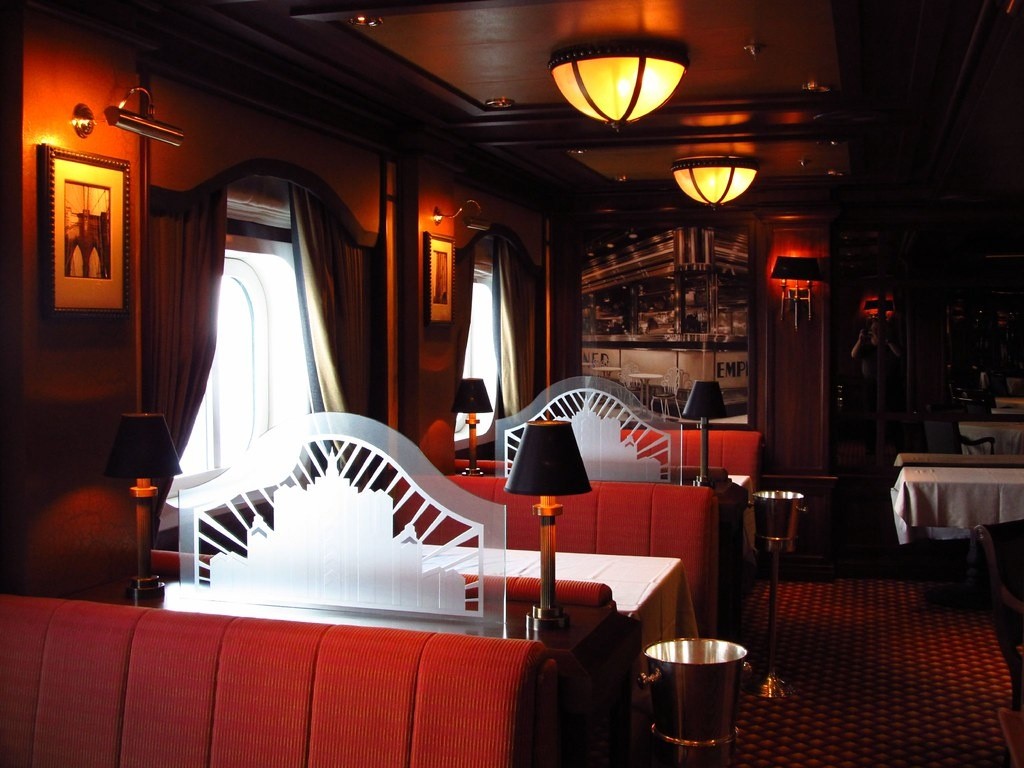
850, 319, 902, 457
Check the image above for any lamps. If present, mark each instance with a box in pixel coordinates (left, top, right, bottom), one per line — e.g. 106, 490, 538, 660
433, 200, 491, 231
770, 255, 828, 333
671, 155, 761, 214
682, 381, 727, 488
102, 415, 181, 599
72, 86, 184, 147
503, 422, 593, 629
548, 42, 690, 133
451, 379, 492, 476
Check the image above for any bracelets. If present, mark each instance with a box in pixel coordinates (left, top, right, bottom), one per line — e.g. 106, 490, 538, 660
887, 338, 893, 345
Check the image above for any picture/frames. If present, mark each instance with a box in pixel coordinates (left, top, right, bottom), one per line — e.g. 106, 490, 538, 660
40, 143, 132, 322
553, 212, 758, 431
423, 230, 457, 328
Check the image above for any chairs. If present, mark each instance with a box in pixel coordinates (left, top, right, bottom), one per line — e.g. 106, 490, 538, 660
618, 361, 644, 403
969, 520, 1024, 768
953, 431, 995, 454
649, 368, 684, 422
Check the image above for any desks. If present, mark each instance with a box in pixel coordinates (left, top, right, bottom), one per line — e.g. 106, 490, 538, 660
418, 542, 698, 697
890, 376, 1024, 611
581, 362, 663, 419
673, 480, 749, 633
728, 474, 756, 560
54, 575, 641, 768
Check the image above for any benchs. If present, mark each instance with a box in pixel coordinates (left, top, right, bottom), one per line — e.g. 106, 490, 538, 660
0, 426, 769, 768
